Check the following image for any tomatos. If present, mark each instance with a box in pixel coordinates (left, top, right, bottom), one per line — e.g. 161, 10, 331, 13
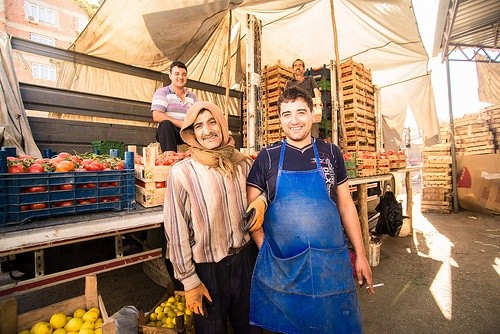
6, 150, 125, 210
343, 150, 407, 169
136, 151, 189, 189
252, 152, 259, 159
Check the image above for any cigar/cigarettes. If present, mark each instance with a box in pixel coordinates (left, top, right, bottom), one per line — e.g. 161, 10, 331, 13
366, 283, 384, 289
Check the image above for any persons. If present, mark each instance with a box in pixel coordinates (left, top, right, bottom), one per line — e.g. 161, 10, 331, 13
246, 87, 375, 334
286, 59, 323, 138
163, 100, 268, 334
150, 62, 198, 153
375, 191, 402, 236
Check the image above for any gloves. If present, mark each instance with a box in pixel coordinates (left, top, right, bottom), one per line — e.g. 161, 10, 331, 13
242, 195, 268, 234
185, 283, 213, 316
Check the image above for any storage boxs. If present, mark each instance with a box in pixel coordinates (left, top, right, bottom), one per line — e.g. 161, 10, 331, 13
242, 55, 500, 212
0, 142, 182, 235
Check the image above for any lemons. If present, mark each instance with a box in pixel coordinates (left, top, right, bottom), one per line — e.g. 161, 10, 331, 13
146, 295, 192, 328
18, 307, 104, 334
